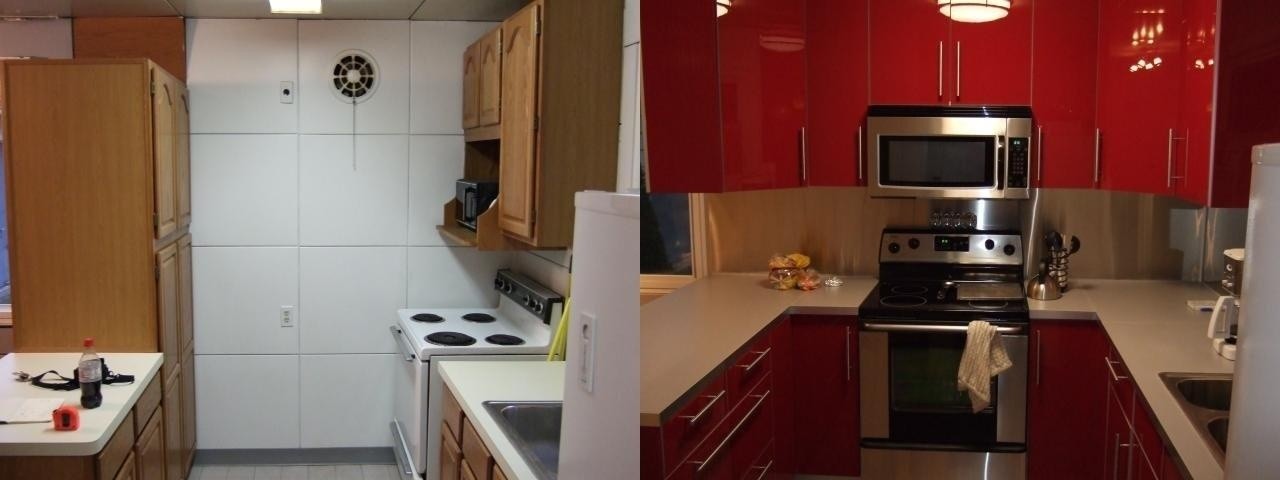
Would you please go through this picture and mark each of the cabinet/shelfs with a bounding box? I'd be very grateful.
[1,351,166,480]
[1029,0,1100,188]
[790,313,860,479]
[0,56,197,480]
[868,1,1035,104]
[1111,344,1187,479]
[1028,317,1113,479]
[809,0,869,188]
[641,313,790,479]
[641,0,811,190]
[461,27,501,140]
[438,380,511,480]
[1100,0,1280,209]
[502,1,625,252]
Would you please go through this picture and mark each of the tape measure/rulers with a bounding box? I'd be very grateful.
[52,404,80,431]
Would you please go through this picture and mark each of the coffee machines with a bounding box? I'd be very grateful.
[1208,244,1251,359]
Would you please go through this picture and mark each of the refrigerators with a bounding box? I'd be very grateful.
[554,191,640,480]
[1225,144,1279,478]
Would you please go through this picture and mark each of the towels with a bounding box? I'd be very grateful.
[957,320,1013,414]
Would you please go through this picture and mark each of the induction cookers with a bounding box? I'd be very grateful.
[859,272,1029,321]
[396,295,554,362]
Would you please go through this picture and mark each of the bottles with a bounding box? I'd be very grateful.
[78,337,102,409]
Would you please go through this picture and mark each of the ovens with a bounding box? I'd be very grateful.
[862,325,1027,480]
[392,328,559,480]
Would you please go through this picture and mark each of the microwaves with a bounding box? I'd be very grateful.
[869,107,1032,200]
[456,179,501,233]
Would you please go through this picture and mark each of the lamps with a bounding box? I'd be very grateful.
[934,1,1011,23]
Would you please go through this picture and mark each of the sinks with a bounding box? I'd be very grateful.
[1158,372,1233,415]
[481,400,562,478]
[1195,415,1229,472]
